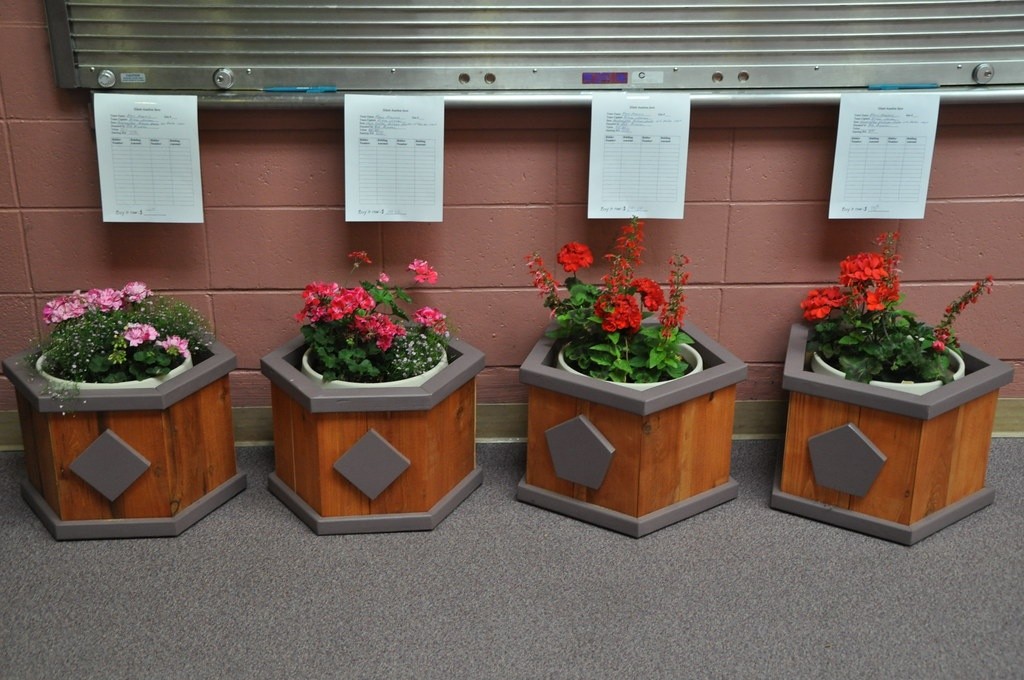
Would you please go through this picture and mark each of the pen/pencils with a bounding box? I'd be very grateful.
[259,86,339,94]
[868,82,939,90]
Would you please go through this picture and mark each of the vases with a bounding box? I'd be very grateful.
[33,329,195,391]
[557,337,705,391]
[809,336,968,396]
[299,332,448,389]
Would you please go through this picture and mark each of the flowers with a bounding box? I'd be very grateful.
[15,281,217,381]
[526,213,696,387]
[298,250,455,384]
[801,231,994,388]
